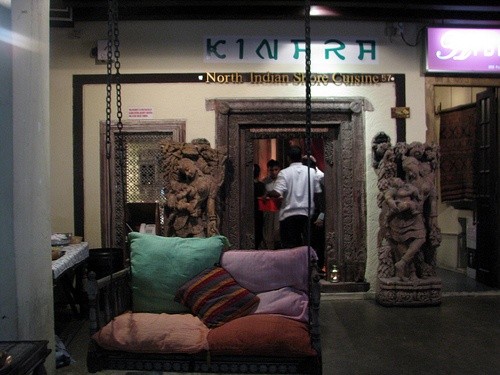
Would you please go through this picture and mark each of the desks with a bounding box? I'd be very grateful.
[52,241,89,321]
[0,340,52,375]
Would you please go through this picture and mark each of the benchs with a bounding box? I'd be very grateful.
[86,266,322,375]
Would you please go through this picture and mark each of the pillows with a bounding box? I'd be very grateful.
[175,264,260,328]
[93,311,210,354]
[128,232,230,314]
[207,315,317,357]
[221,246,318,322]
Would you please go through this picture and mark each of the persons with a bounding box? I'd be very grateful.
[254,145,326,275]
[166,158,219,237]
[386,156,436,281]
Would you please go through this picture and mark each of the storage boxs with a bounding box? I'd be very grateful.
[258,197,278,212]
